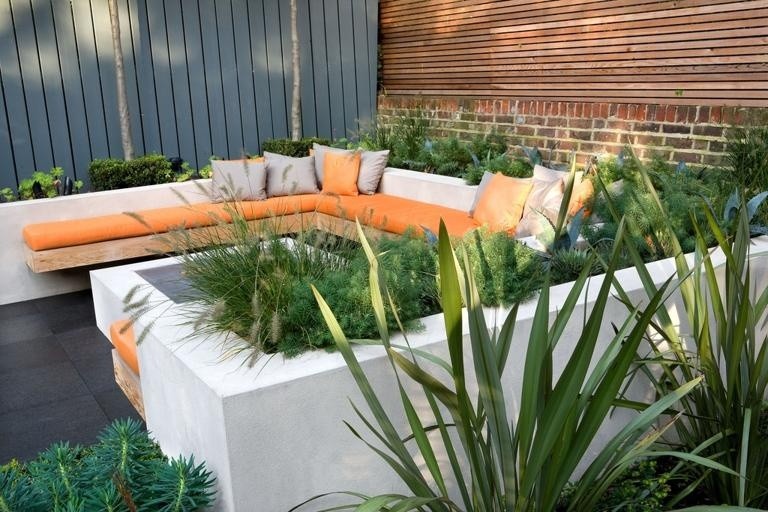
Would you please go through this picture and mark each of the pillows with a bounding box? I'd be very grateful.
[206,141,390,204]
[469,164,624,253]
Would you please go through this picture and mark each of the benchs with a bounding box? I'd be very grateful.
[19,165,505,282]
